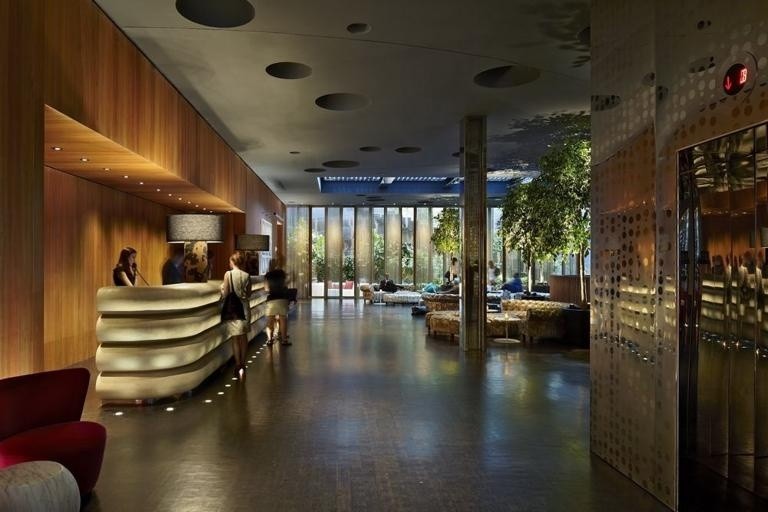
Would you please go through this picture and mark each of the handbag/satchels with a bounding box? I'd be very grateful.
[222,290,246,323]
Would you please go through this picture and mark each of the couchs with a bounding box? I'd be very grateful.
[422,292,496,312]
[360,285,426,304]
[502,299,570,344]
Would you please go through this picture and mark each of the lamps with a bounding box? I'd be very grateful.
[234,234,269,276]
[166,214,224,283]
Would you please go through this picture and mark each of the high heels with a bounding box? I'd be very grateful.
[240,360,247,374]
[232,364,241,378]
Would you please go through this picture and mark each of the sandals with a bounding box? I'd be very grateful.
[267,340,273,346]
[281,340,292,345]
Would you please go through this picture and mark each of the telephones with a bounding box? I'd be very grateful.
[132,262,138,268]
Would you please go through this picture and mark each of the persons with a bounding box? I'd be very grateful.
[488,267,502,309]
[163,247,187,285]
[111,247,141,288]
[501,273,523,293]
[220,251,252,380]
[379,273,394,292]
[449,257,459,281]
[487,260,497,285]
[265,258,293,345]
[176,252,200,282]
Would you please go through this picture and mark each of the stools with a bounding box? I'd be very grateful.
[0,461,81,512]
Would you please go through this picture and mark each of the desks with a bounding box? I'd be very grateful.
[426,310,522,344]
[383,294,421,306]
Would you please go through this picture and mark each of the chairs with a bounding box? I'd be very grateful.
[0,368,106,500]
